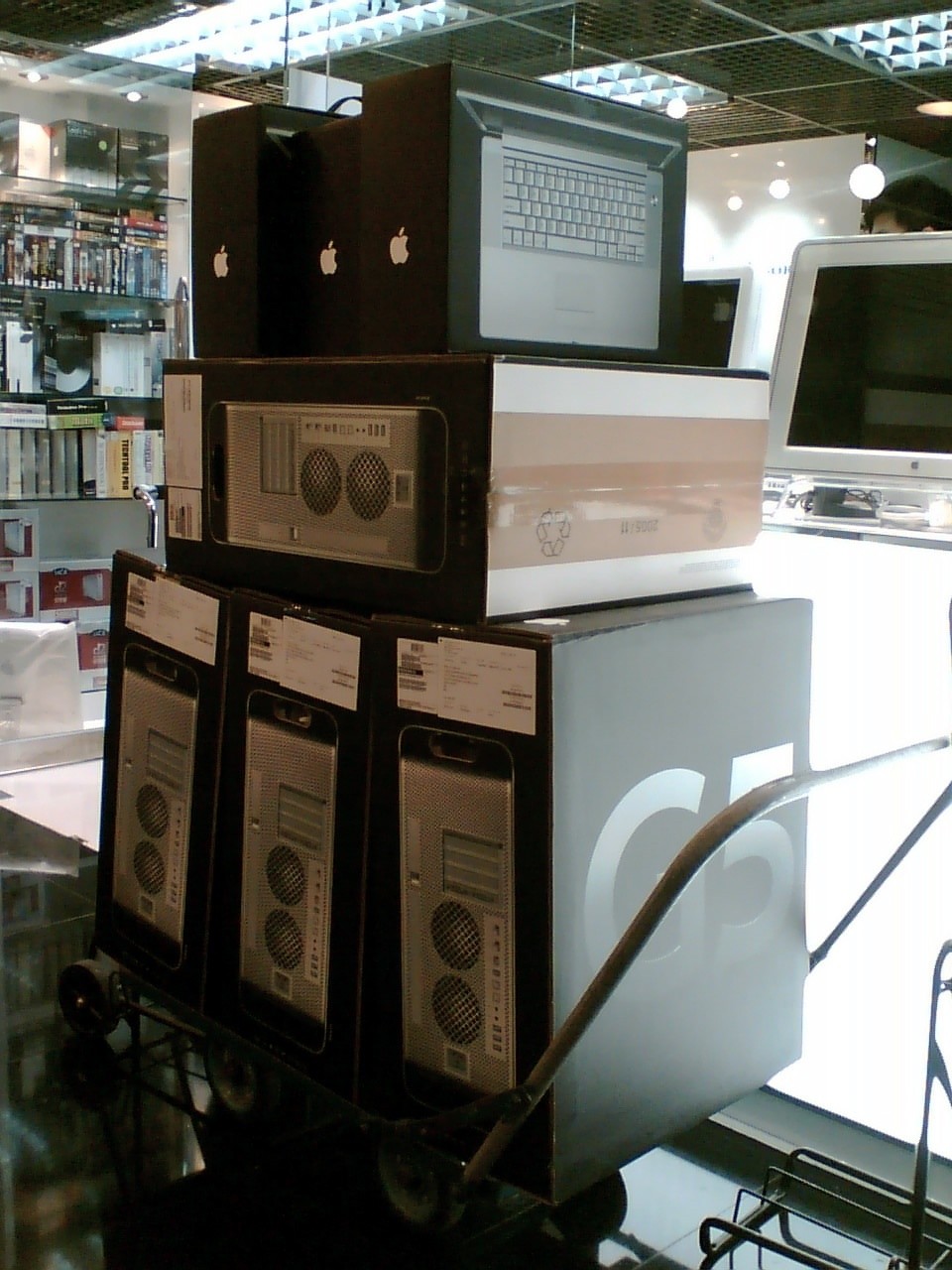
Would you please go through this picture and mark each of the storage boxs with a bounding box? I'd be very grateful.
[0,509,114,693]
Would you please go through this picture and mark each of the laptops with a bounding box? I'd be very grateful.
[455,87,683,359]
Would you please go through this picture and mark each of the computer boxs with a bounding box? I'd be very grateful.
[90,354,820,1201]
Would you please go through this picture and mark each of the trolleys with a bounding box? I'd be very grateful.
[54,735,951,1269]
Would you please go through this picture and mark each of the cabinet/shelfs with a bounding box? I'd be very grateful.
[1,175,187,504]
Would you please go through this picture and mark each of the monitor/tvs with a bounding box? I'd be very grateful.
[763,231,952,521]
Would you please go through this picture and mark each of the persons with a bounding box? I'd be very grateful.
[863,175,952,234]
